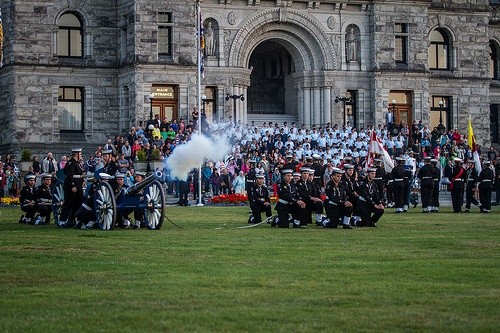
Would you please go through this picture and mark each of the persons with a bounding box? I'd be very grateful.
[0,109,500,229]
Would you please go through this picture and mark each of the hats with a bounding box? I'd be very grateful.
[394,157,406,164]
[102,149,112,155]
[114,174,125,179]
[454,158,491,165]
[99,172,111,180]
[25,173,37,181]
[306,156,312,160]
[255,173,265,179]
[367,168,377,171]
[331,164,354,173]
[424,156,438,162]
[71,148,83,152]
[312,154,324,160]
[136,170,147,176]
[261,154,266,157]
[286,156,293,159]
[249,160,255,165]
[346,157,352,161]
[42,174,53,180]
[293,168,315,176]
[282,169,294,176]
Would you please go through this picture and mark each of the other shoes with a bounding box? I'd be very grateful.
[387,202,492,214]
[18,214,141,229]
[247,214,378,229]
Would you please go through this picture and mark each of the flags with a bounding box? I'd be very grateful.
[196,8,206,80]
[468,118,482,176]
[370,130,394,172]
